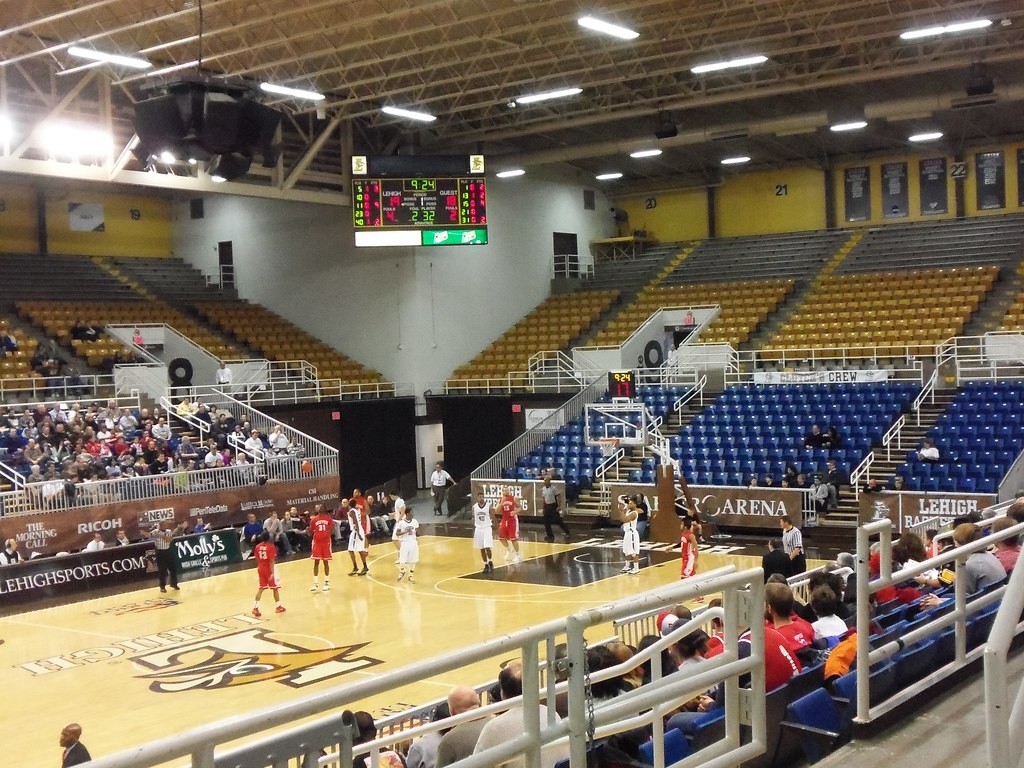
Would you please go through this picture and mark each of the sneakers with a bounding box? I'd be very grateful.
[252,608,261,616]
[628,569,639,574]
[311,584,319,591]
[322,583,329,591]
[621,567,630,572]
[276,606,285,612]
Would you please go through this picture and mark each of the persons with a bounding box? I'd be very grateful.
[336,488,1024,768]
[0,516,212,565]
[305,503,335,590]
[664,517,704,604]
[0,328,19,359]
[750,424,843,519]
[526,467,563,480]
[431,462,456,515]
[252,530,286,616]
[30,339,90,396]
[863,479,882,493]
[151,518,180,592]
[892,476,907,490]
[73,320,96,338]
[541,477,571,543]
[244,503,344,556]
[391,490,410,564]
[916,438,939,465]
[497,489,523,561]
[471,492,499,572]
[59,723,91,768]
[620,496,640,575]
[396,506,420,585]
[216,362,232,396]
[0,395,308,509]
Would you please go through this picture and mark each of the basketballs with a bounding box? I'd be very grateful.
[301,463,313,472]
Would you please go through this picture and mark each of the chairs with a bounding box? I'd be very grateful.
[0,261,1024,768]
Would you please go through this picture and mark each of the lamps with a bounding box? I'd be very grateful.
[1002,18,1012,25]
[653,100,679,139]
[507,101,515,107]
[965,61,995,97]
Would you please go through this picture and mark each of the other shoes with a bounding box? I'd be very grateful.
[484,565,489,573]
[358,568,368,575]
[171,585,180,590]
[489,565,493,572]
[397,572,405,581]
[409,577,415,583]
[348,568,358,575]
[161,587,166,592]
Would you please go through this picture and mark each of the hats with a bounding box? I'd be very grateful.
[663,619,688,635]
[657,611,679,633]
